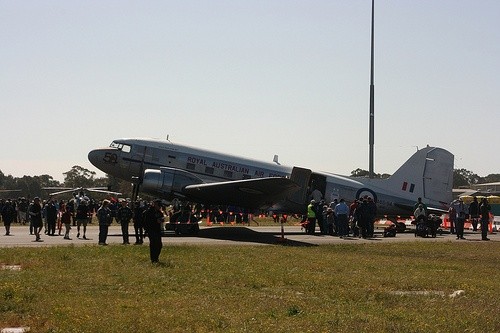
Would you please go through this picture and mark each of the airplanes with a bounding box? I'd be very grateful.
[87,134,454,224]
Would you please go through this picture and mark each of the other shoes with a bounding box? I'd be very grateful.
[134,242,139,244]
[456,236,459,239]
[45,230,62,236]
[64,236,70,239]
[123,242,130,244]
[482,237,490,241]
[77,233,80,237]
[30,232,32,234]
[99,242,107,245]
[139,241,143,245]
[460,237,466,239]
[473,229,477,231]
[36,238,41,241]
[83,236,86,239]
[6,232,10,235]
[307,230,375,239]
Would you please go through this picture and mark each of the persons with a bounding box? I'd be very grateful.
[450,198,469,240]
[478,197,490,241]
[469,197,480,231]
[141,199,166,263]
[413,197,439,238]
[448,202,458,235]
[0,185,379,245]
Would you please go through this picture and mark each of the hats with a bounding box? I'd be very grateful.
[311,200,315,203]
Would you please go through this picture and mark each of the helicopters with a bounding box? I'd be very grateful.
[41,186,124,206]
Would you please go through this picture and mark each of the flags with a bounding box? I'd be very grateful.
[401,181,415,193]
